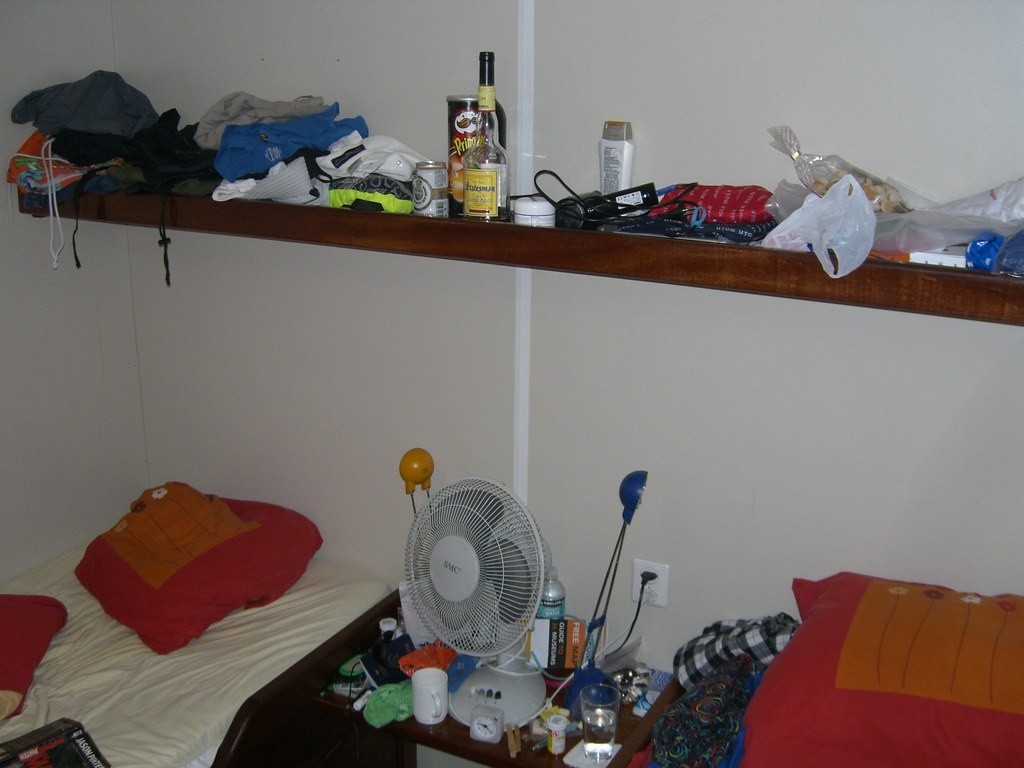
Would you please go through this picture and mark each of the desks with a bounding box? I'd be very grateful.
[313,669,681,768]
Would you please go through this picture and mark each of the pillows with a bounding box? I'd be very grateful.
[0,537,401,767]
[0,593,70,719]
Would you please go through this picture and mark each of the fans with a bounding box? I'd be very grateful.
[405,476,552,736]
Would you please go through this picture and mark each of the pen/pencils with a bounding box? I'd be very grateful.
[531,720,578,753]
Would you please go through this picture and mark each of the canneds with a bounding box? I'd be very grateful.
[412,162,449,218]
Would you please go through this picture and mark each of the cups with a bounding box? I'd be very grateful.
[581,685,621,763]
[412,668,448,725]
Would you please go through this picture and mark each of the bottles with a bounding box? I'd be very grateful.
[463,52,509,220]
[535,567,565,620]
[380,618,397,641]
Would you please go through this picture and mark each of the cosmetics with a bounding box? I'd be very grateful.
[352,688,375,713]
[515,197,555,226]
[597,121,633,196]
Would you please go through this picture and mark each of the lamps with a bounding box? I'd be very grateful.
[562,470,649,718]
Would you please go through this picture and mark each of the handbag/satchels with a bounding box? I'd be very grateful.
[760,174,877,278]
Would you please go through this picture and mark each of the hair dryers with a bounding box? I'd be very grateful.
[553,182,660,230]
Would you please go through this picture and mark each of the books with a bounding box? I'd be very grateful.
[520,618,582,671]
[0,717,112,768]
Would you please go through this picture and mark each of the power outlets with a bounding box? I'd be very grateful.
[630,559,669,607]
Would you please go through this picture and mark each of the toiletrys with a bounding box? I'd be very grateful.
[598,122,633,194]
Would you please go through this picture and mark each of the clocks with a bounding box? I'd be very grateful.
[469,705,505,745]
